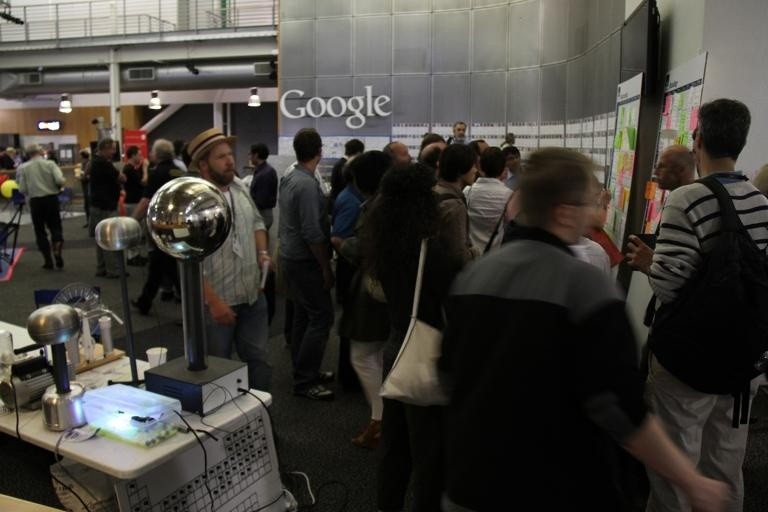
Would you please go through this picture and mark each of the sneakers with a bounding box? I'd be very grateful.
[313,369,334,381]
[293,382,335,400]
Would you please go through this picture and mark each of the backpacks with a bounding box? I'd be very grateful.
[644,177,767,395]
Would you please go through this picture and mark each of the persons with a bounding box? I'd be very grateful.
[432,143,740,510]
[186,127,767,356]
[446,121,470,145]
[1,139,190,312]
[352,161,462,511]
[623,96,766,510]
[326,149,411,453]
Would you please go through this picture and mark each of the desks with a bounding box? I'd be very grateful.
[1,319,280,511]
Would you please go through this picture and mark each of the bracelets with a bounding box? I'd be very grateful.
[257,248,268,256]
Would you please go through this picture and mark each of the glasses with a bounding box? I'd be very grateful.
[591,183,607,206]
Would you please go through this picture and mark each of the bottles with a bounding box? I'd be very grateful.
[97,315,112,358]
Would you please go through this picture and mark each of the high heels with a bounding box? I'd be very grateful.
[351,420,383,448]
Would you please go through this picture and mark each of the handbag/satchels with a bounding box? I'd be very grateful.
[377,317,455,408]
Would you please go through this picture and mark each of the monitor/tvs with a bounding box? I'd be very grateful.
[619,0,660,98]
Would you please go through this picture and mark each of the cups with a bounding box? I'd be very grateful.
[145,347,168,368]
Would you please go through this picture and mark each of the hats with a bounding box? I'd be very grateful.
[187,128,239,174]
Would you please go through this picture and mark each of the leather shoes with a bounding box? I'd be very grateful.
[131,296,150,315]
[126,257,144,266]
[161,291,175,300]
[106,271,130,278]
[137,255,148,262]
[54,250,63,268]
[97,271,106,276]
[42,264,53,271]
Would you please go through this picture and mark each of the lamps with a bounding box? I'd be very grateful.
[58,93,71,113]
[246,87,260,107]
[148,90,162,109]
